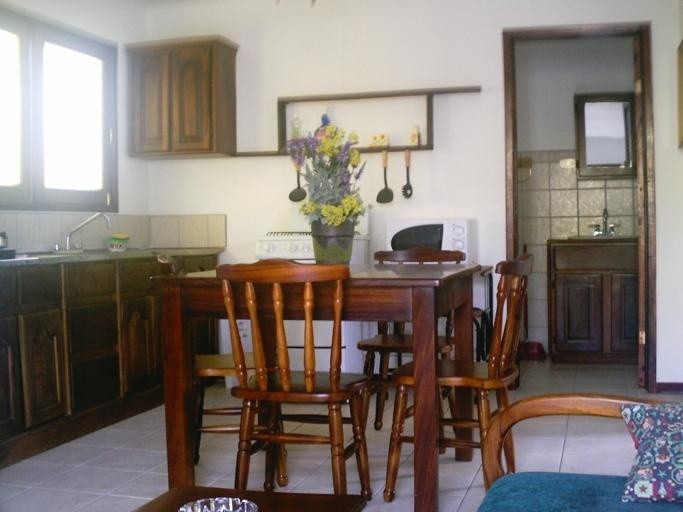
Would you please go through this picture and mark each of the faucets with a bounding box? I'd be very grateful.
[602,209,609,236]
[65,209,112,251]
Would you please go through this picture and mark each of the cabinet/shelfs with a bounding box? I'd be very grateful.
[1,263,72,464]
[546,240,640,364]
[65,261,123,435]
[122,255,220,421]
[123,36,241,159]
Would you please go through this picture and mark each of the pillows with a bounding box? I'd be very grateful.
[620,403,683,505]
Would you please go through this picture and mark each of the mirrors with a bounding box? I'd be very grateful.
[573,92,636,180]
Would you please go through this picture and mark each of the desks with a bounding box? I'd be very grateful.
[143,262,486,512]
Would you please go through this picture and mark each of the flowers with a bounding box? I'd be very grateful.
[290,123,372,224]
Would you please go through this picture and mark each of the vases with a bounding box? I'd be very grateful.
[308,213,357,267]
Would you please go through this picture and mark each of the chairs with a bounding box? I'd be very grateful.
[474,391,683,512]
